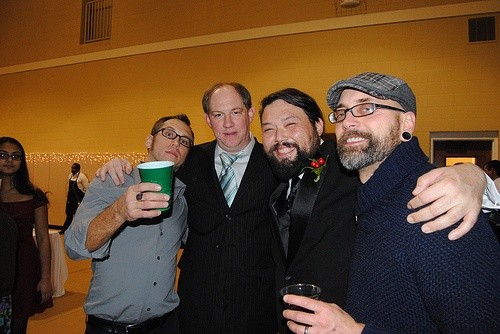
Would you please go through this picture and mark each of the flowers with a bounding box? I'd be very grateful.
[301,154,330,182]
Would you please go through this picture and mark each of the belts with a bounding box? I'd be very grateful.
[88,311,173,334]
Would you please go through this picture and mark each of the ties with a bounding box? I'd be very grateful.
[219,153,239,208]
[285,175,301,216]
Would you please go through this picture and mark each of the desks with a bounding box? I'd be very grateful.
[33,229,61,296]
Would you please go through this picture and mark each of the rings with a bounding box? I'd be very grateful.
[136,192,143,200]
[304,325,308,334]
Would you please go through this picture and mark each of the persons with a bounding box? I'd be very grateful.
[281,74,500,334]
[258,87,500,334]
[0,138,53,334]
[59,164,91,235]
[67,114,195,334]
[483,159,500,195]
[96,83,260,334]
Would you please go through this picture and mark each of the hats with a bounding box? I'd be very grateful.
[327,72,416,116]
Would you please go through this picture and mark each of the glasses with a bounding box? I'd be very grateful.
[328,103,407,123]
[0,153,21,160]
[153,128,193,148]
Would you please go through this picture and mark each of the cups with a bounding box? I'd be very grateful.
[137,161,175,212]
[280,283,322,327]
